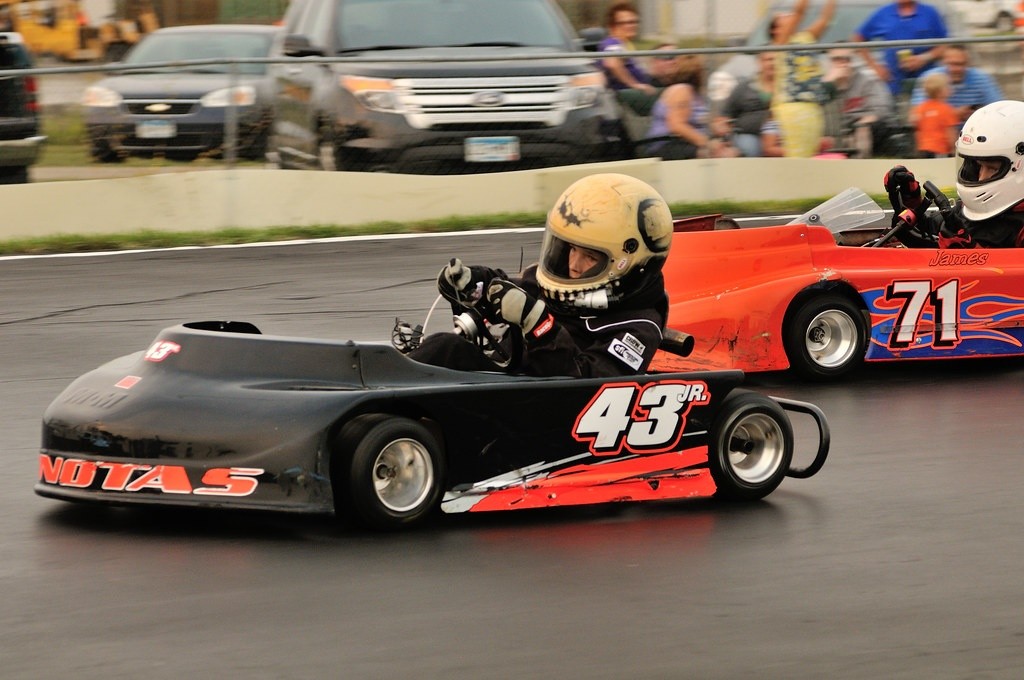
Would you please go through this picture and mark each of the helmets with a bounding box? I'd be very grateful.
[536,174,673,303]
[955,98,1024,223]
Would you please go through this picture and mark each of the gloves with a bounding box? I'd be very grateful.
[437,257,482,308]
[487,276,544,338]
[939,219,974,250]
[885,166,920,205]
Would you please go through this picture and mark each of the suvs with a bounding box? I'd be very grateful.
[270,1,637,176]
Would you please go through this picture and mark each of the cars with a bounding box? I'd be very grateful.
[78,22,281,163]
[962,0,1023,35]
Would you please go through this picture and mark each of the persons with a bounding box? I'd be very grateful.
[596,1,998,159]
[884,100,1024,248]
[405,174,673,379]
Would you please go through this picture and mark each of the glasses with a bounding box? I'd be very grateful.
[612,19,640,24]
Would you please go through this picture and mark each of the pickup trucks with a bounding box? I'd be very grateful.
[2,30,50,186]
